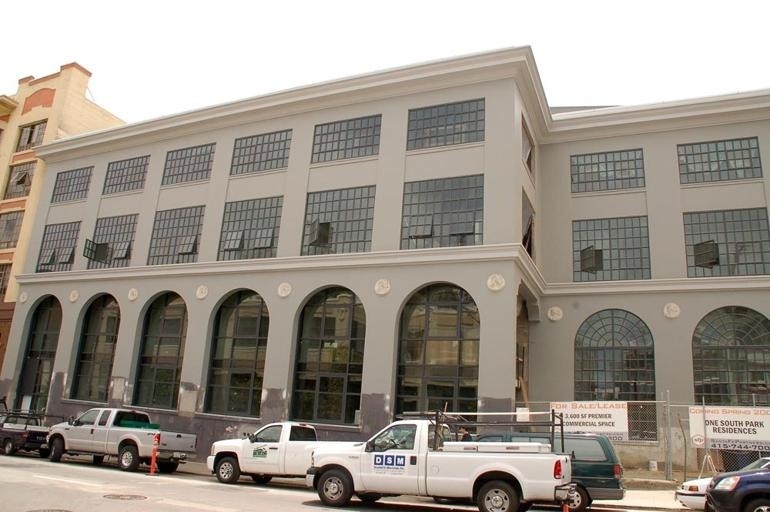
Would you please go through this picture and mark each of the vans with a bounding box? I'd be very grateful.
[466,430,623,512]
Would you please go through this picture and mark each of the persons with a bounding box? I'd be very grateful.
[459,426,472,441]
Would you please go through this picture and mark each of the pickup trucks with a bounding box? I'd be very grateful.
[45,408,197,472]
[207,421,366,485]
[307,414,577,512]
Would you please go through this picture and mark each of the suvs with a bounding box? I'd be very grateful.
[0,408,50,458]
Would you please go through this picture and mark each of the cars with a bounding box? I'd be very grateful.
[676,458,770,512]
